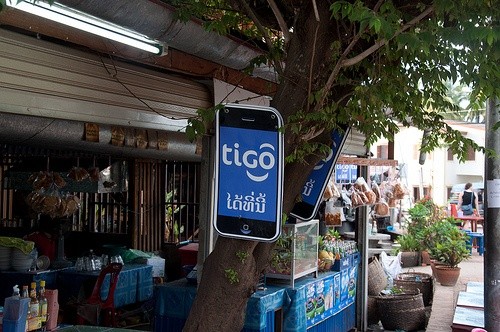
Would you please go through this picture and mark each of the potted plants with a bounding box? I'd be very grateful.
[397,198,469,286]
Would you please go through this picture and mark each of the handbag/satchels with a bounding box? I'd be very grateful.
[461,204,473,216]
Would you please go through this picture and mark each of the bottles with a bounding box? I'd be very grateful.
[13,280,48,332]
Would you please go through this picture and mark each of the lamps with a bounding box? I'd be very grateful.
[6,0,168,56]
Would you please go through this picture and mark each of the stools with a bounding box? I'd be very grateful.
[462,230,483,256]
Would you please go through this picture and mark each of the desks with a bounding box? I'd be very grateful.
[455,217,485,248]
[0,257,365,332]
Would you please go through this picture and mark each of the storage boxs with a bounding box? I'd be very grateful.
[332,252,361,271]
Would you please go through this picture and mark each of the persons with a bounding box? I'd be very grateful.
[23,214,60,259]
[456,183,480,228]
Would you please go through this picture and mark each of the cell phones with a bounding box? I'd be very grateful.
[213,102,283,243]
[288,118,354,222]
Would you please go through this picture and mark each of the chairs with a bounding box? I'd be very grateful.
[65,262,122,326]
